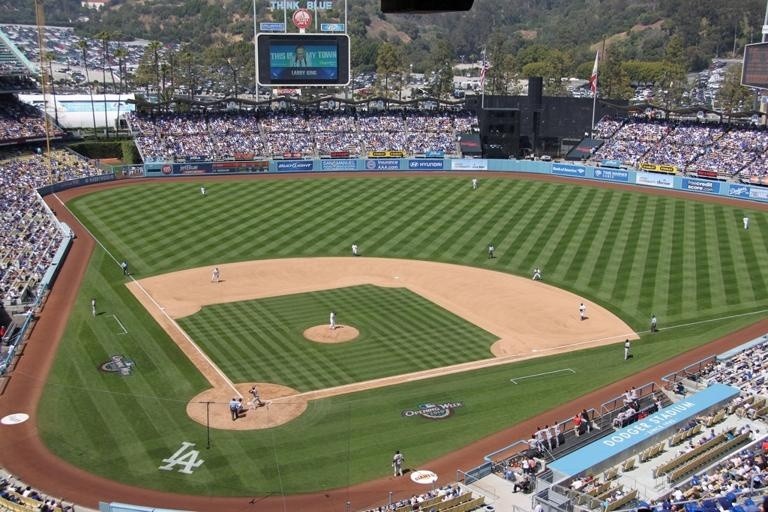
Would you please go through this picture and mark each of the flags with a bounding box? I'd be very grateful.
[480,51,494,87]
[587,53,601,95]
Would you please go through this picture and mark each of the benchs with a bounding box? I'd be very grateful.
[394,490,486,511]
[567,342,768,512]
[0,486,64,512]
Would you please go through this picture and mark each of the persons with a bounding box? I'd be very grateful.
[330,311,337,329]
[578,303,585,321]
[472,177,478,190]
[531,267,543,281]
[590,115,767,196]
[211,267,220,282]
[237,398,244,414]
[487,244,496,259]
[742,216,749,230]
[200,186,205,196]
[1,468,76,511]
[351,241,358,255]
[229,398,239,421]
[292,46,311,68]
[121,260,128,275]
[248,384,263,411]
[650,315,656,332]
